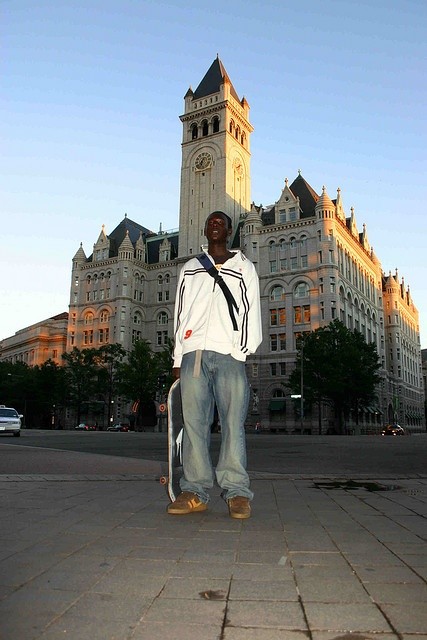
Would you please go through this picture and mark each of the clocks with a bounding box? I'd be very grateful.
[194,151,213,172]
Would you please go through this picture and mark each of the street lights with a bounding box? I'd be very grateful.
[7,374,27,428]
[108,400,114,427]
[145,340,171,395]
[301,328,331,434]
[53,404,56,429]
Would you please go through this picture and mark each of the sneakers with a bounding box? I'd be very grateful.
[167,491,209,514]
[229,495,251,519]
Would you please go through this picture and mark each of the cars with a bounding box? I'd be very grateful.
[75,423,96,430]
[107,423,128,432]
[0,405,23,436]
[380,424,404,436]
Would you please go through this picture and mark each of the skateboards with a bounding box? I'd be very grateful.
[159,378,184,502]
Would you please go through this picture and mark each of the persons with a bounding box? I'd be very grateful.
[166,210,262,519]
